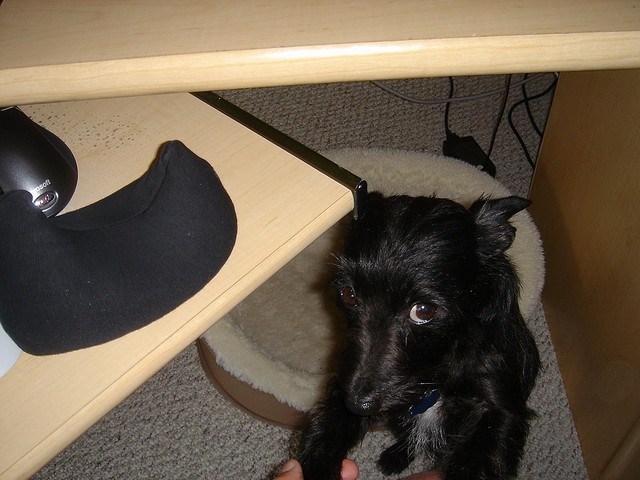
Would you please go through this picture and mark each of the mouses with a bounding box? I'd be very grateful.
[0,106,78,219]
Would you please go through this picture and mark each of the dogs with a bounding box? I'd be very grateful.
[298,190,543,480]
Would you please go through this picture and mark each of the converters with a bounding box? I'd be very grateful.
[442,136,496,180]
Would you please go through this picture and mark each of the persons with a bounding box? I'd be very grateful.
[271,458,359,479]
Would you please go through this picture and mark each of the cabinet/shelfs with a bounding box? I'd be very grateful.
[0,0,639,479]
